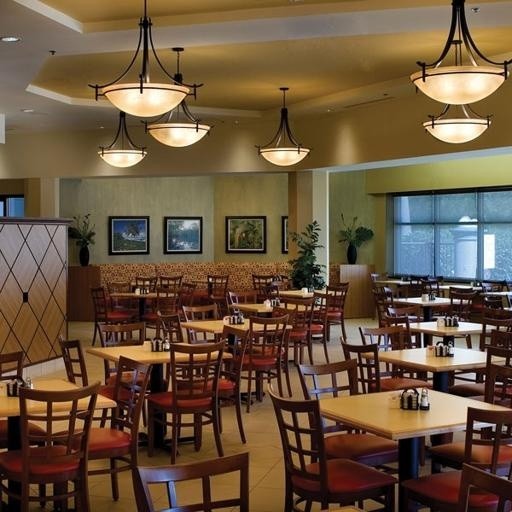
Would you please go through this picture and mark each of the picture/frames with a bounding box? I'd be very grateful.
[280,213,289,255]
[162,214,203,255]
[225,215,268,254]
[106,214,150,256]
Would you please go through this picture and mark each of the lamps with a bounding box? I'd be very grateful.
[146,46,216,148]
[256,88,313,167]
[96,108,147,168]
[90,0,204,120]
[406,2,512,103]
[420,98,492,146]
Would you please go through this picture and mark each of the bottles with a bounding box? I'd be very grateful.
[142,334,172,353]
[388,386,434,413]
[434,313,464,329]
[224,307,247,326]
[262,298,283,308]
[426,340,455,359]
[6,375,36,398]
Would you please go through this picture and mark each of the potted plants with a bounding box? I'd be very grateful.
[68,214,96,265]
[336,211,374,265]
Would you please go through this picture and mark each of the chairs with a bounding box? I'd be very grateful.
[1,273,512,512]
